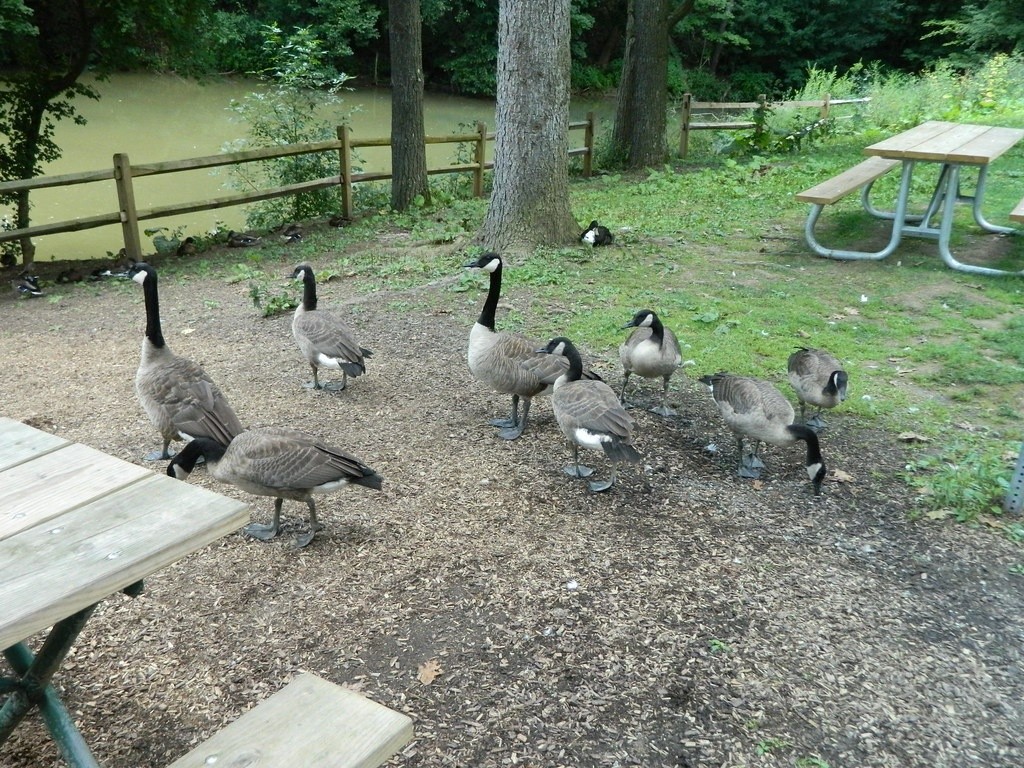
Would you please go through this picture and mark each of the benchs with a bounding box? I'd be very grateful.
[161,672,415,768]
[795,155,904,205]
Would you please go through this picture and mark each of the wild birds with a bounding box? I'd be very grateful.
[165,426,383,550]
[285,264,373,392]
[101,262,243,468]
[580,220,614,248]
[459,252,609,440]
[618,308,684,417]
[698,372,826,500]
[534,336,647,493]
[786,345,849,432]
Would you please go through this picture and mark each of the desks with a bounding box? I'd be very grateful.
[0,415,252,767]
[795,119,1024,279]
